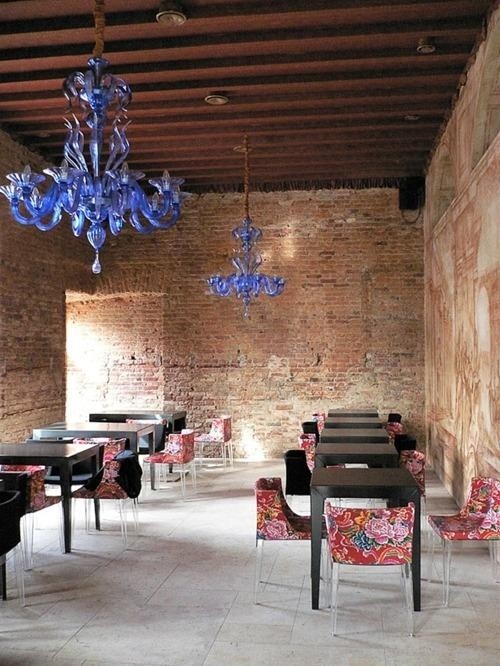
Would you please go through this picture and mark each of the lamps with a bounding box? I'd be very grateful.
[2,1,184,279]
[207,135,286,319]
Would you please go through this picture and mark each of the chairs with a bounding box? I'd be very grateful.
[143,429,198,498]
[425,471,498,607]
[192,416,234,470]
[255,476,327,609]
[382,406,428,495]
[323,499,417,639]
[0,463,67,571]
[67,436,141,545]
[282,407,342,510]
[0,490,27,607]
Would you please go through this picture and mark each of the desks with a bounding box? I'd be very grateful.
[0,442,101,553]
[316,406,402,505]
[30,407,189,505]
[309,467,424,612]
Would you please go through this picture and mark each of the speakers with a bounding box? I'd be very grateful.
[399,177,425,209]
